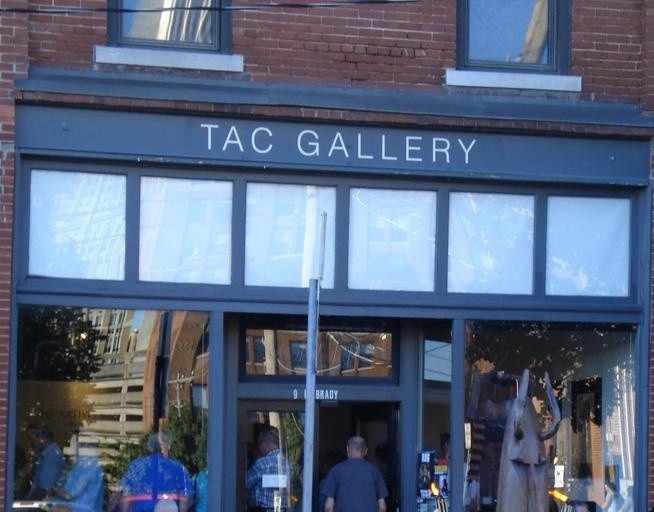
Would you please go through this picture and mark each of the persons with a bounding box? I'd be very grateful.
[26,423,104,512]
[107,432,208,512]
[601,483,633,512]
[319,436,388,512]
[245,427,295,512]
